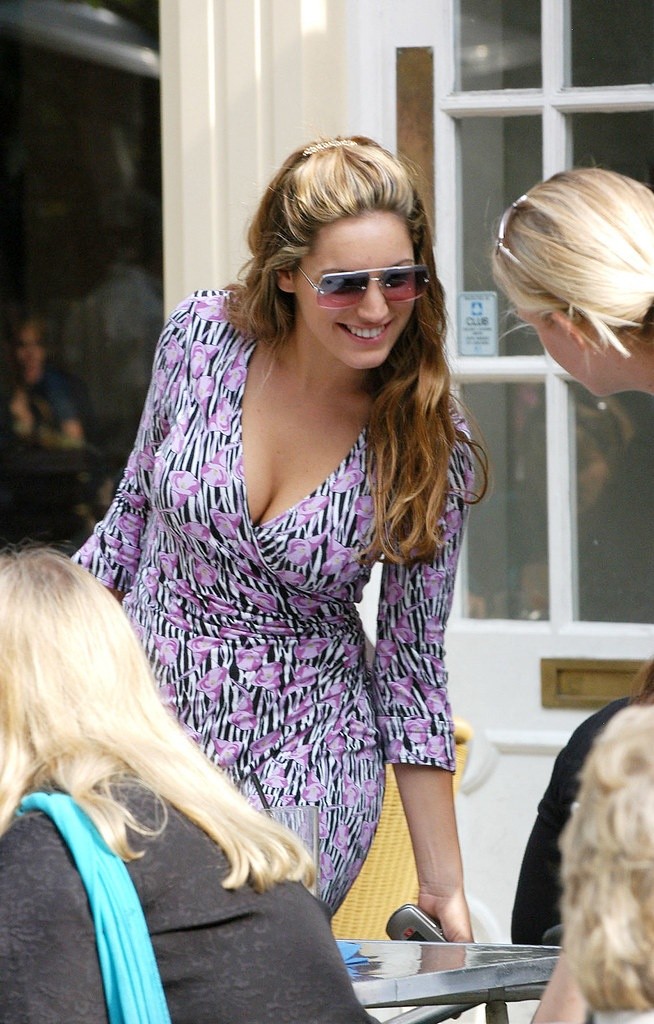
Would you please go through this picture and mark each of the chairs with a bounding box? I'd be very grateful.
[330,722,470,938]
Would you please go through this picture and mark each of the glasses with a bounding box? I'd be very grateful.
[293,251,431,310]
[492,195,585,326]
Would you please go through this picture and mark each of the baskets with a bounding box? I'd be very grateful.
[331,711,472,939]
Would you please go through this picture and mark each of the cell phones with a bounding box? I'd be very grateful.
[385,904,448,942]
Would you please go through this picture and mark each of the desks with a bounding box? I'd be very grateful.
[336,938,562,1024]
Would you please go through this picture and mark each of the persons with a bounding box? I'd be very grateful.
[494,167,654,393]
[513,384,603,509]
[530,704,654,1024]
[511,662,654,946]
[0,547,379,1024]
[0,306,115,548]
[68,137,473,942]
